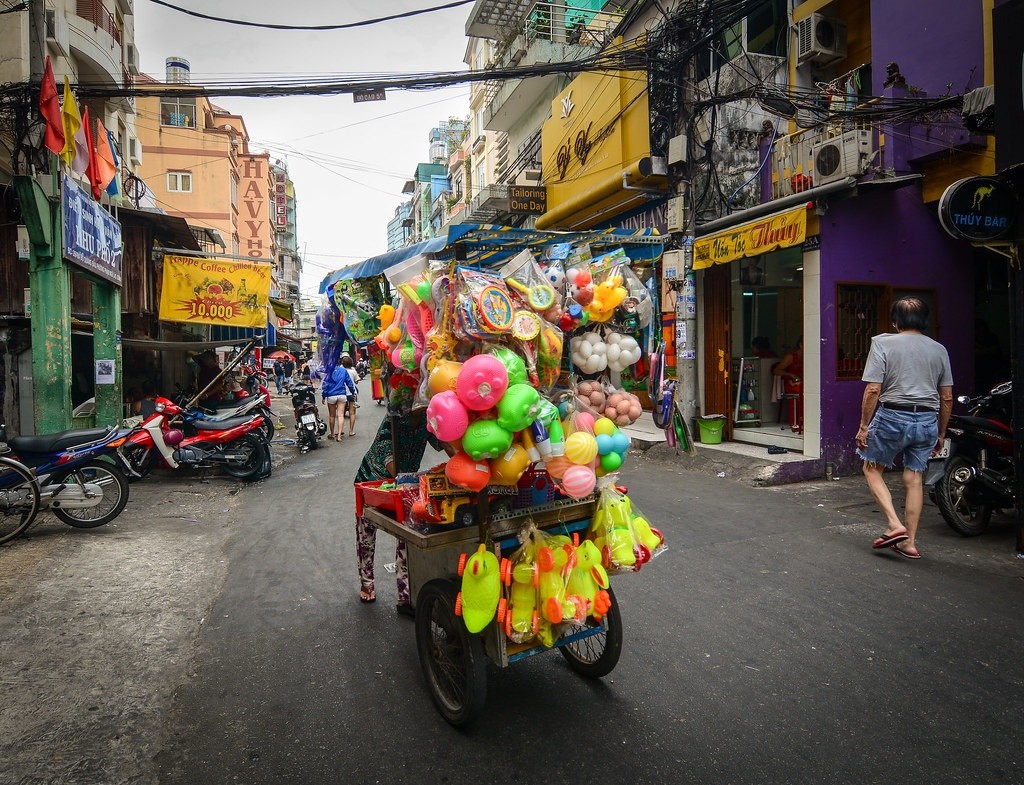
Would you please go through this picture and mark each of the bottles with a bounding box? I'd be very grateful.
[666,427,676,447]
[732,359,758,420]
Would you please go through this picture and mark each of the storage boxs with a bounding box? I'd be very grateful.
[73,416,95,429]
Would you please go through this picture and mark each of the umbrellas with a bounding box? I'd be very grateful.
[265,350,296,363]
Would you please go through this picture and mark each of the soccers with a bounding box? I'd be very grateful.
[543,267,565,290]
[567,283,581,298]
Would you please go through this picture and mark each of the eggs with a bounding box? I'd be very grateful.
[569,332,641,374]
[577,381,643,425]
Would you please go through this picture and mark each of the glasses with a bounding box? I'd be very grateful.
[892,321,896,328]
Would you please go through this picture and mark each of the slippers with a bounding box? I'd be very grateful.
[396,603,416,618]
[872,533,910,549]
[889,543,922,559]
[360,586,377,603]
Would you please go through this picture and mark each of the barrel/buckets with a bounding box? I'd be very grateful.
[697,420,724,445]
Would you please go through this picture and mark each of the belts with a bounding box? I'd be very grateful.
[882,401,936,412]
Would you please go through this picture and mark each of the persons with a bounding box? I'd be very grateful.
[187,350,225,410]
[335,351,365,436]
[353,394,455,619]
[772,332,803,428]
[321,355,356,442]
[853,295,954,560]
[272,354,294,395]
[301,357,310,384]
[132,379,164,421]
[225,376,271,408]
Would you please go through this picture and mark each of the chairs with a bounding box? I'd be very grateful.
[771,362,798,425]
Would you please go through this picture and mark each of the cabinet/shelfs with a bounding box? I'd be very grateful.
[732,356,762,428]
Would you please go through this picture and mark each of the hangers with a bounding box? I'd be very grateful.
[815,67,861,94]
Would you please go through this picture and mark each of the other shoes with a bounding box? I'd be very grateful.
[336,437,344,442]
[349,432,356,436]
[328,434,336,440]
[335,432,345,435]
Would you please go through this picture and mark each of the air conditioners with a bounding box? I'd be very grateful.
[812,130,872,187]
[798,12,848,63]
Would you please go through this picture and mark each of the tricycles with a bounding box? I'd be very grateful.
[361,491,626,715]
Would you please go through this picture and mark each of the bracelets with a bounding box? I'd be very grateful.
[203,392,210,398]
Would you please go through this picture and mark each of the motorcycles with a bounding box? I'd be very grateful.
[0,425,129,544]
[111,390,273,481]
[289,385,327,451]
[246,358,268,388]
[923,378,1024,534]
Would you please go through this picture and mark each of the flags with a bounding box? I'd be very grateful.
[39,55,120,201]
